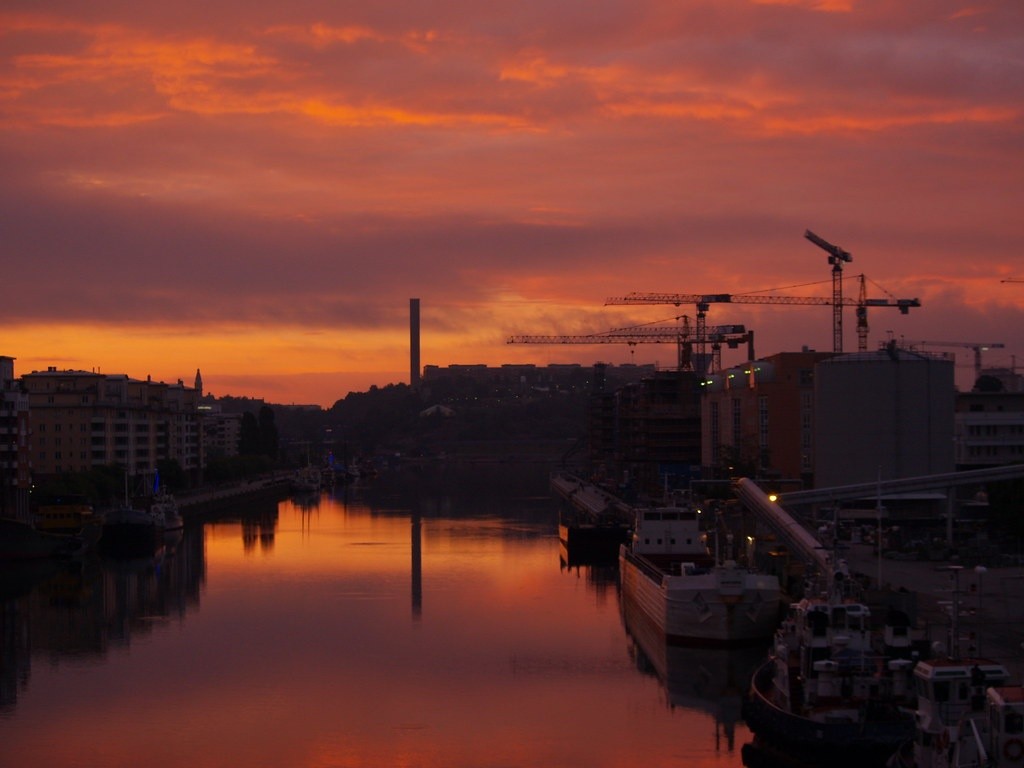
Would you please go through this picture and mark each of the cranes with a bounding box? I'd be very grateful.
[805,228,853,354]
[506,313,755,374]
[878,340,1005,383]
[605,273,926,352]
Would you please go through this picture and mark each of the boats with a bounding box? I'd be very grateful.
[291,462,321,492]
[117,471,183,528]
[620,504,782,645]
[749,593,921,765]
[874,565,1024,768]
